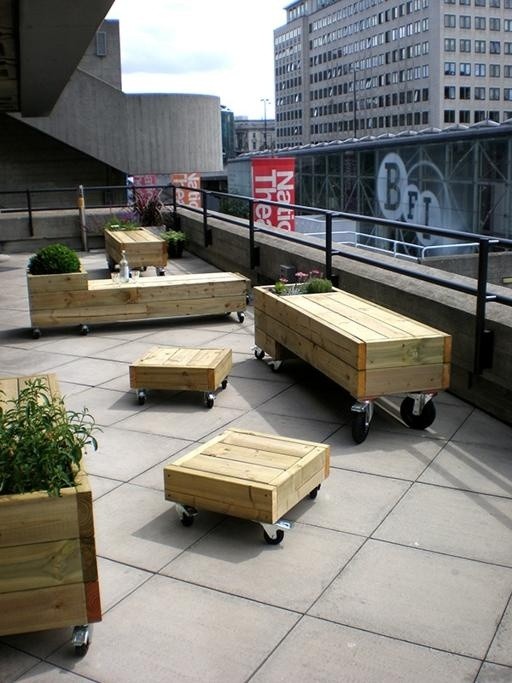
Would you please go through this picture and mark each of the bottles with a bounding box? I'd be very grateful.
[120,251,129,283]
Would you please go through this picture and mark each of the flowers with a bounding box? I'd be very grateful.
[274,270,333,294]
[103,209,141,230]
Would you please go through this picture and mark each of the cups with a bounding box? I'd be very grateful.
[130,271,139,284]
[111,272,120,286]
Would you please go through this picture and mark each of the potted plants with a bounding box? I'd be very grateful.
[0,373,104,657]
[26,243,88,291]
[159,228,187,258]
